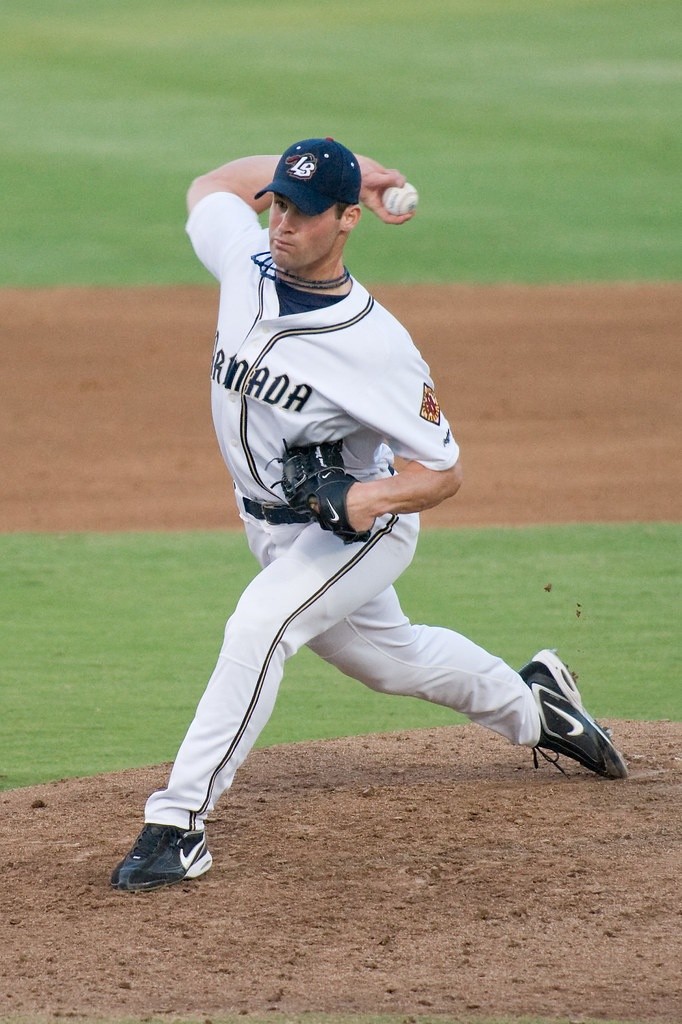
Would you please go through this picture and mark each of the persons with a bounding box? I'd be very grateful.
[111,141,632,895]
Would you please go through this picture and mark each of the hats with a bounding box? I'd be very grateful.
[252,135,363,216]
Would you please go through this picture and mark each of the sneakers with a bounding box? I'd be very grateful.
[111,822,213,890]
[514,648,628,782]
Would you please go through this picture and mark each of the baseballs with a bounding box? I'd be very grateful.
[382,180,419,216]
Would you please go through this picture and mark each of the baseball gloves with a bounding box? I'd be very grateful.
[263,439,371,547]
